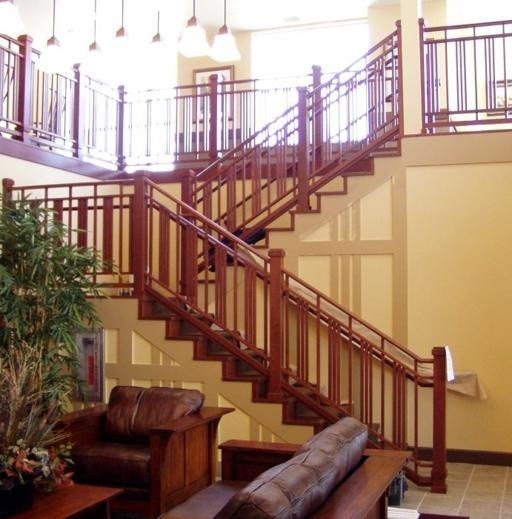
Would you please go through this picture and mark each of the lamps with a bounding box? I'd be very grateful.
[0,0,240,79]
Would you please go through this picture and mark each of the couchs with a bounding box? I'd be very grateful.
[46,397,235,518]
[156,438,414,519]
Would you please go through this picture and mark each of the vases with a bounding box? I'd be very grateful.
[0,477,34,519]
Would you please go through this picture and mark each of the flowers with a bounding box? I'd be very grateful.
[0,438,78,493]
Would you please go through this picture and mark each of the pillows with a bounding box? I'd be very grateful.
[103,385,204,443]
[212,417,369,519]
